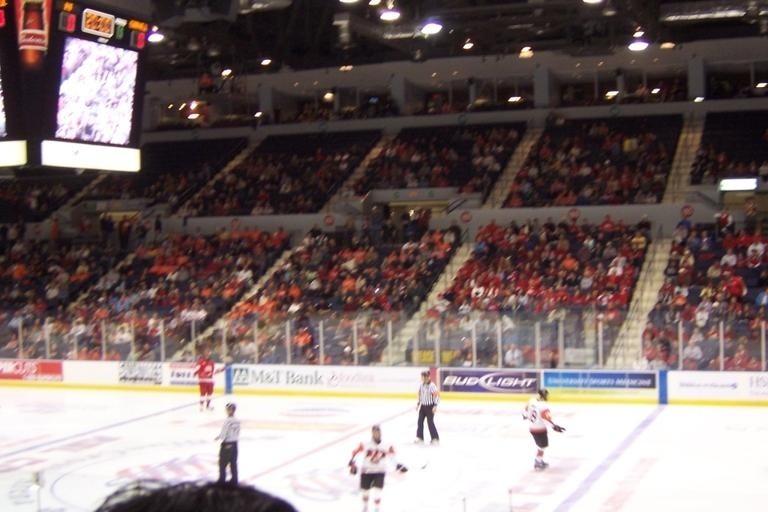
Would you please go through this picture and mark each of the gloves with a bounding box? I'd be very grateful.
[552,425,566,432]
[395,463,408,474]
[348,461,359,474]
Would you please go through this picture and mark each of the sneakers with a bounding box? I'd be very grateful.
[534,458,549,470]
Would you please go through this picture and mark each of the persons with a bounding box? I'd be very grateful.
[346,425,394,512]
[214,404,240,485]
[413,373,441,444]
[1,122,766,370]
[522,389,566,470]
[88,473,296,512]
[192,350,225,411]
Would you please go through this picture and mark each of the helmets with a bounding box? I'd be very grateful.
[372,424,380,433]
[226,402,236,410]
[537,387,549,394]
[421,370,431,377]
